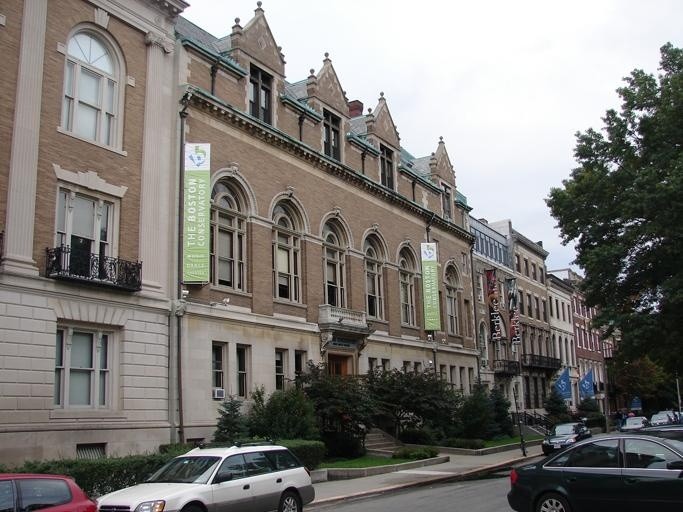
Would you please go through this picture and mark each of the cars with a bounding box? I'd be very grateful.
[507,410,683,512]
[0,473,97,512]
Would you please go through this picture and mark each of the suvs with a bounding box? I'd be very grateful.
[95,440,316,512]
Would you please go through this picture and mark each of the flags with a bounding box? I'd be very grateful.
[554,368,571,400]
[579,369,595,400]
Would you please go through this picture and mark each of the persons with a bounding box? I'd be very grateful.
[618,410,635,427]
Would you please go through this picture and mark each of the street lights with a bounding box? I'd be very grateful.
[432,342,438,381]
